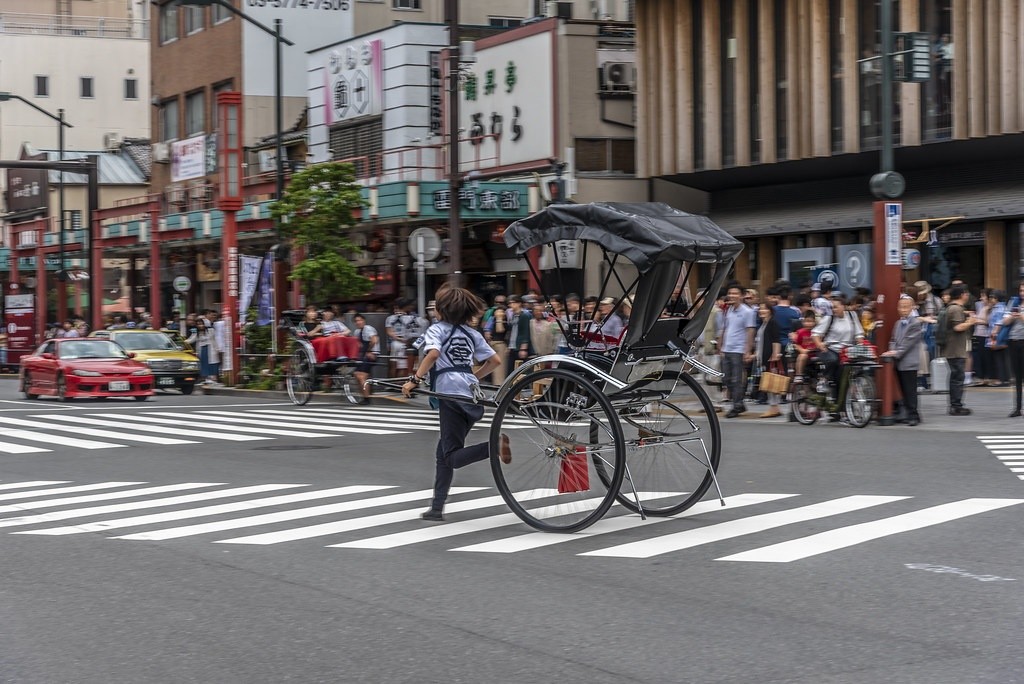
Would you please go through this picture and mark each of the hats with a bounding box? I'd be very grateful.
[914,281,932,295]
[829,291,841,296]
[811,283,824,293]
[600,297,617,305]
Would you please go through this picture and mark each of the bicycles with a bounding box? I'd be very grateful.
[786,340,886,429]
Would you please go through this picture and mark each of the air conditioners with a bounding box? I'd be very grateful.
[601,61,637,91]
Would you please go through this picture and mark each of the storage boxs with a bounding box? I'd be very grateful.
[838,345,877,365]
[758,371,790,395]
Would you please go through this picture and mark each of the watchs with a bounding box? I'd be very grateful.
[409,376,419,385]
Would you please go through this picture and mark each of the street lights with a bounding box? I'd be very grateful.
[0,91,75,331]
[151,1,295,264]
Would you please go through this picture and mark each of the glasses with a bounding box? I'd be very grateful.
[506,301,519,305]
[495,301,506,305]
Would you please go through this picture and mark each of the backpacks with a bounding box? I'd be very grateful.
[935,304,959,346]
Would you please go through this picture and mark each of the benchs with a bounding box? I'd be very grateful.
[505,316,698,421]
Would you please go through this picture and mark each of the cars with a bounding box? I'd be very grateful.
[87,328,203,397]
[17,337,157,402]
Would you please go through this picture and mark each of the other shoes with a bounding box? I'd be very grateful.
[423,510,442,521]
[499,434,511,464]
[910,418,920,426]
[759,411,781,417]
[726,405,746,418]
[949,407,969,415]
[1010,410,1021,417]
[794,375,804,383]
[816,381,827,394]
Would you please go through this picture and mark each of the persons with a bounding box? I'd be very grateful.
[879,298,918,423]
[692,277,884,422]
[161,309,224,386]
[386,297,437,397]
[45,314,92,338]
[471,289,653,417]
[352,313,380,404]
[104,312,153,330]
[902,281,1024,417]
[667,286,689,316]
[301,306,360,360]
[402,287,511,519]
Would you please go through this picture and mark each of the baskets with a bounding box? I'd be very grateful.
[845,345,879,359]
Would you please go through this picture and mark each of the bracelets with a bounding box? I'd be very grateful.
[416,374,425,380]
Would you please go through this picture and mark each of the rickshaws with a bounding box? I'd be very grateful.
[277,308,410,404]
[358,203,745,537]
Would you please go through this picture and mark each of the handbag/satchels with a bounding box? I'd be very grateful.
[759,359,791,395]
